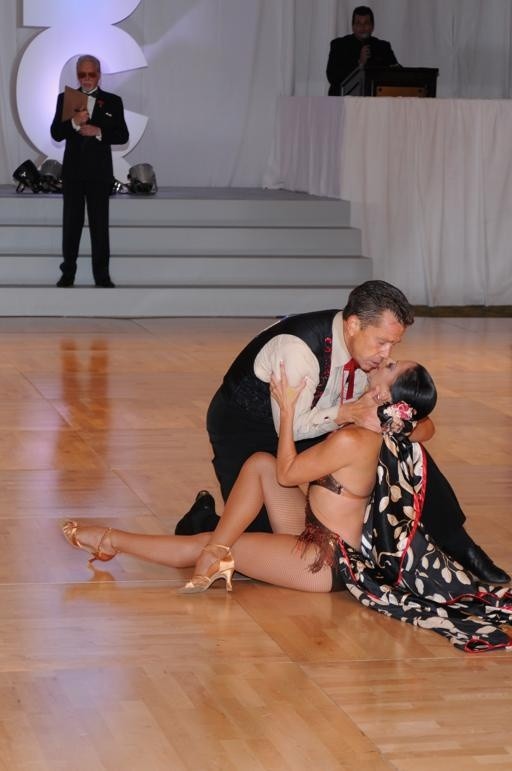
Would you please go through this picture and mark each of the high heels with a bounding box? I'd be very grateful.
[58,519,120,563]
[170,543,235,594]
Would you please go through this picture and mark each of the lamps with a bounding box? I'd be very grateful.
[12,159,157,195]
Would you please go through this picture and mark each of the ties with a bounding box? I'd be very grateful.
[342,359,359,400]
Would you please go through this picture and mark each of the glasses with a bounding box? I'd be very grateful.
[78,72,96,78]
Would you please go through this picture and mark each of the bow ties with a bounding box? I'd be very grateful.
[83,91,98,98]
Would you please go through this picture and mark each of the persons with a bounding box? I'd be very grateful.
[58,359,512,654]
[50,55,129,289]
[175,279,415,536]
[326,5,403,96]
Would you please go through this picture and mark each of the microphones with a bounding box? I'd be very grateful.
[362,32,369,46]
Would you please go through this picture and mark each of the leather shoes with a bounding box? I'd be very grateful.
[176,490,215,535]
[444,545,511,583]
[56,273,116,288]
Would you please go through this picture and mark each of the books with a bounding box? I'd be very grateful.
[62,85,88,128]
